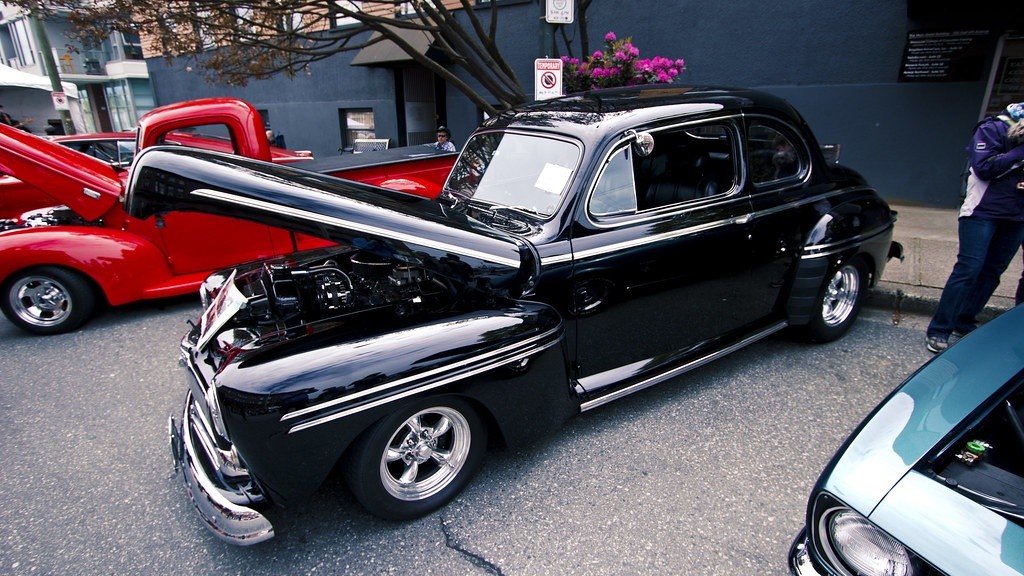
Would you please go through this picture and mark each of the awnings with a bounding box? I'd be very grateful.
[350,13,454,66]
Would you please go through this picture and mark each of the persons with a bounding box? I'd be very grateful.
[0,105,13,126]
[423,126,456,152]
[772,133,800,180]
[925,102,1024,353]
[695,151,718,197]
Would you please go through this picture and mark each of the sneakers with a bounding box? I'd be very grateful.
[925,336,949,352]
[951,325,977,337]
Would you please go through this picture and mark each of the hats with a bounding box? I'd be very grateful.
[435,125,449,132]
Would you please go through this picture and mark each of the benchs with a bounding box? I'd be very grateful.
[338,139,392,154]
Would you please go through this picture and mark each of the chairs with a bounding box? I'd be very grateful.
[603,147,720,207]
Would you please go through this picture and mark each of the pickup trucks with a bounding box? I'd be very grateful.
[0,98,460,339]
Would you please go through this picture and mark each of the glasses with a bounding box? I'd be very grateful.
[437,135,447,137]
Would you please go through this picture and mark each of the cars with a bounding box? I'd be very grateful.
[123,87,902,548]
[0,130,314,176]
[789,302,1023,576]
